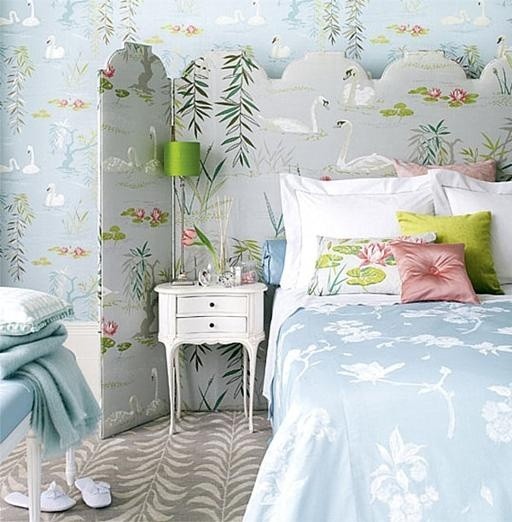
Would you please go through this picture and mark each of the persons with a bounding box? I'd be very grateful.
[201,272,207,283]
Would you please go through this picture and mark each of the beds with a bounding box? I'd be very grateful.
[242,234,511,522]
[0,349,77,522]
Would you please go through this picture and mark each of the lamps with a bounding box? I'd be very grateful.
[162,141,201,286]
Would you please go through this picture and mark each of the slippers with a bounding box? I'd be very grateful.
[4,481,76,511]
[75,476,111,508]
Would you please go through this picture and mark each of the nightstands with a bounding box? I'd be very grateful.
[154,281,267,435]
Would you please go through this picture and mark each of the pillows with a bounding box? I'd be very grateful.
[279,172,434,292]
[396,209,506,296]
[0,287,75,338]
[306,231,438,295]
[428,169,512,285]
[391,241,481,306]
[392,156,497,183]
